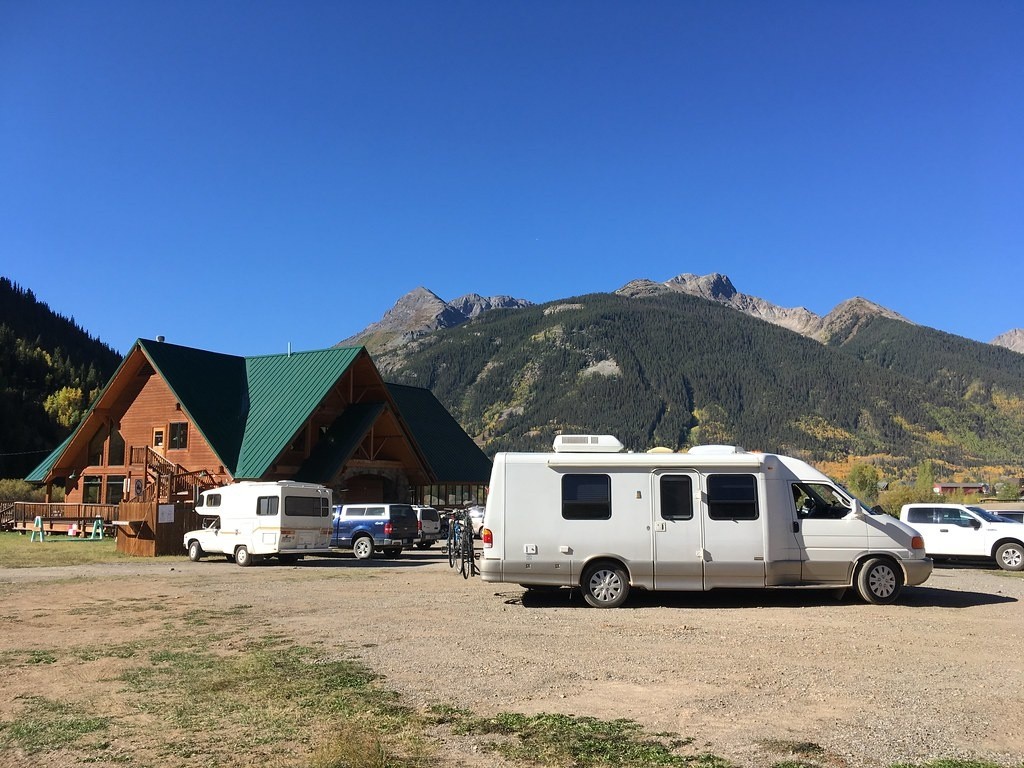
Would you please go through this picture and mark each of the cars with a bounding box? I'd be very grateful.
[438,507,484,540]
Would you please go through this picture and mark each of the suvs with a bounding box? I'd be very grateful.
[900,504,1024,571]
[408,505,441,549]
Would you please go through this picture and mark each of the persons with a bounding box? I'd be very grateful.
[793,484,801,503]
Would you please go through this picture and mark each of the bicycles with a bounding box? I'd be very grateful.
[445,507,477,580]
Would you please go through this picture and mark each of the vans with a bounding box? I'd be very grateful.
[182,479,336,566]
[478,435,933,609]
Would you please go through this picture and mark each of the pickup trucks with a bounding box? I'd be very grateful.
[330,503,417,561]
